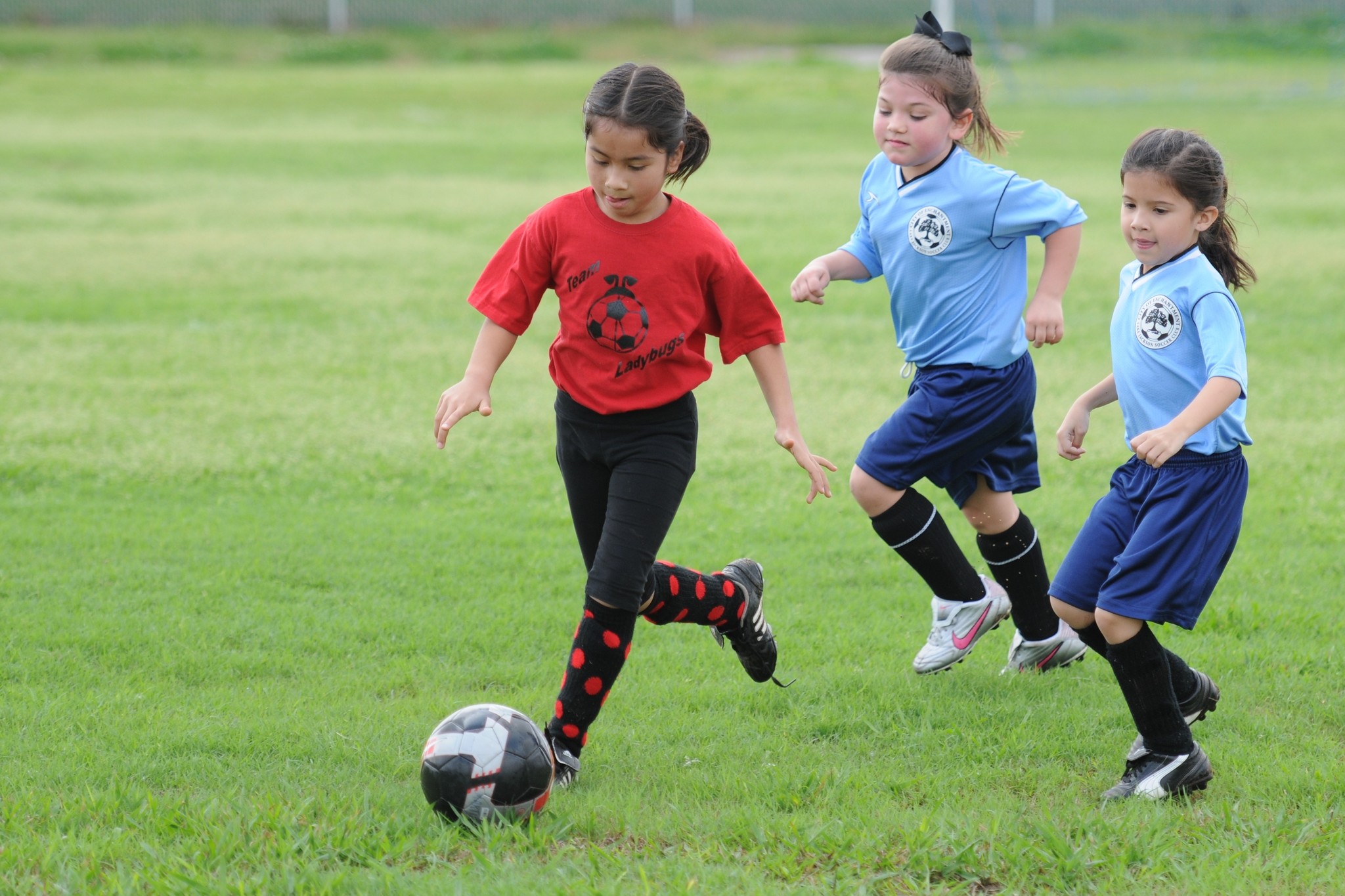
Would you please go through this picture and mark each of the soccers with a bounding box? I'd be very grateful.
[419,703,556,837]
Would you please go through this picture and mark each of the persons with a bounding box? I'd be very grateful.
[790,11,1094,673]
[1055,130,1261,804]
[433,64,837,791]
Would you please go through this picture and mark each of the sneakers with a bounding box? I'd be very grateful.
[1126,665,1221,773]
[542,722,581,790]
[999,620,1089,679]
[914,575,1014,675]
[1101,741,1215,806]
[709,558,797,689]
